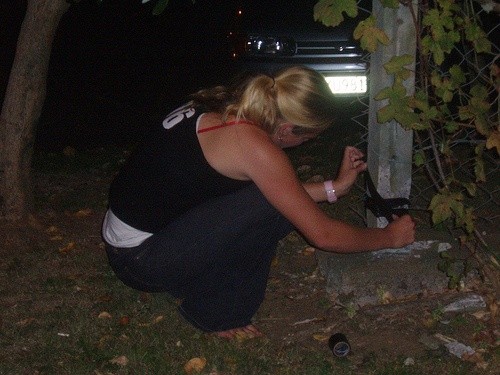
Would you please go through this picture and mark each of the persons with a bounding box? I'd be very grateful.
[99,66,417,340]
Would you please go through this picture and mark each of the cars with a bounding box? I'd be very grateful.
[233,1,425,107]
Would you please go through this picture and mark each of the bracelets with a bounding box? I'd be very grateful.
[324,179,338,204]
